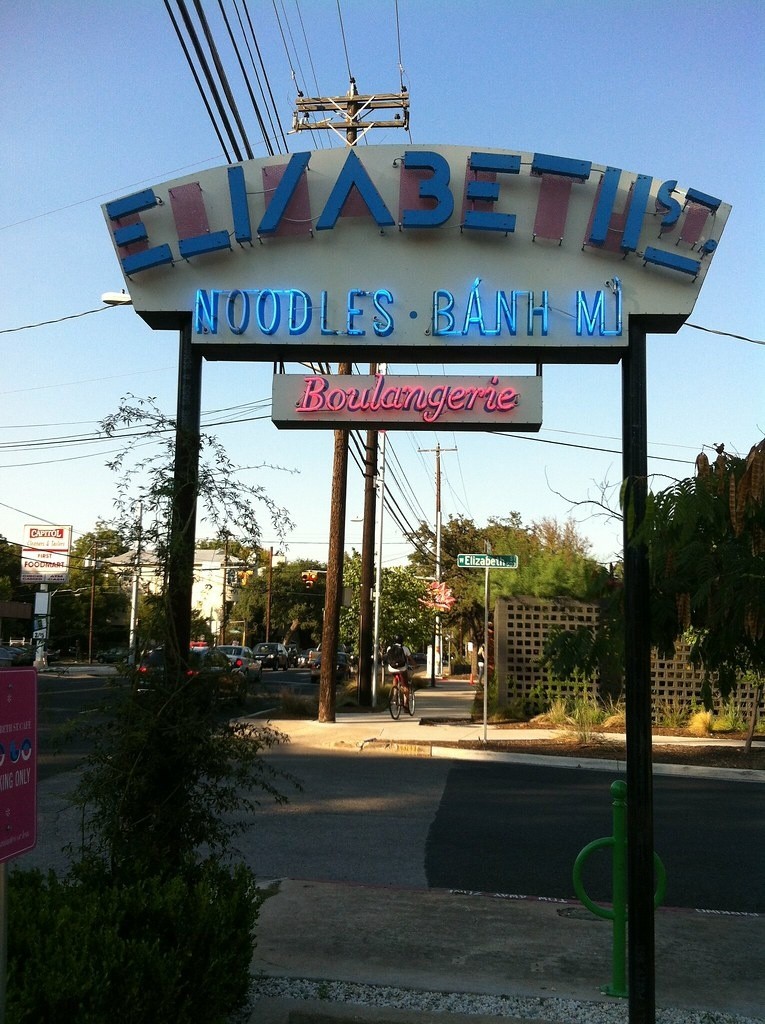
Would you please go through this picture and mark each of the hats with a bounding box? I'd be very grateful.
[394,635,403,643]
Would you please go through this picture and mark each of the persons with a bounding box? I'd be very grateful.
[387,635,417,710]
[478,646,485,684]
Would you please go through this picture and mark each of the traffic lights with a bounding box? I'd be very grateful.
[302,572,318,589]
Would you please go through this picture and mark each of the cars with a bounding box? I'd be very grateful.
[133,640,352,705]
[0,646,56,665]
[96,647,133,664]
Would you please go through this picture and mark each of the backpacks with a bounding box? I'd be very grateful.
[385,645,407,669]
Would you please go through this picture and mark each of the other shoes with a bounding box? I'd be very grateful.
[404,703,408,708]
[390,698,397,702]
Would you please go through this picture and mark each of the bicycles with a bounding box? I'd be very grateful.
[388,665,421,721]
